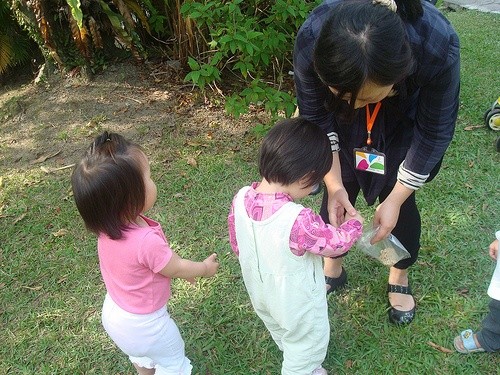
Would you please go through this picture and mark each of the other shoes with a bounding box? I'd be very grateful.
[311,366,327,375]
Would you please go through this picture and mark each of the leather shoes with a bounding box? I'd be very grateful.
[386,284,416,326]
[324,266,347,295]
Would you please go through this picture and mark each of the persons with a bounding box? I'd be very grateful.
[455,231,500,354]
[71,130,219,375]
[227,116,366,375]
[293,0,460,329]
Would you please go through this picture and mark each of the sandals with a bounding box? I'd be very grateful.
[454,329,486,353]
